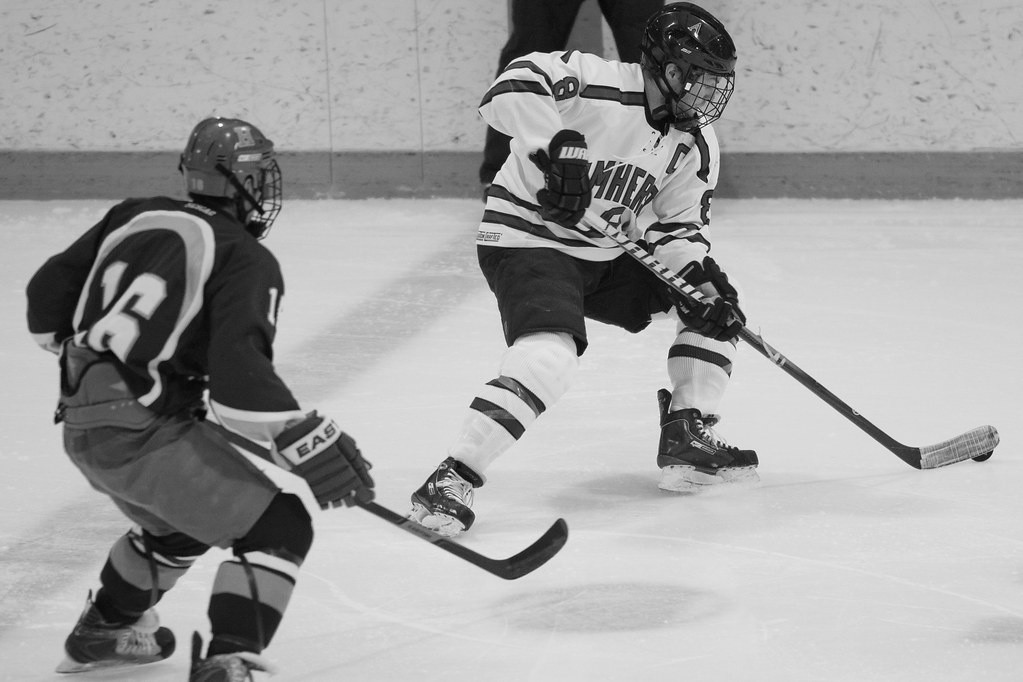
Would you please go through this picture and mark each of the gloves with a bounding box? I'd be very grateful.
[535,130,591,228]
[669,256,746,340]
[271,409,375,512]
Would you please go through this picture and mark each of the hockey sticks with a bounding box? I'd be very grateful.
[582,207,1001,471]
[203,416,571,581]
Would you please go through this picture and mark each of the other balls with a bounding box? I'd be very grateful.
[972,450,993,462]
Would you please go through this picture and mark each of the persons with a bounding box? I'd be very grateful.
[26,115,376,681]
[406,1,758,533]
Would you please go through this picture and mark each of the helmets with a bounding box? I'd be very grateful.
[639,1,738,133]
[181,117,280,239]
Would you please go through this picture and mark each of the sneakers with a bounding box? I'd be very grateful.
[189,631,271,682]
[407,456,483,539]
[656,388,759,492]
[55,588,176,675]
